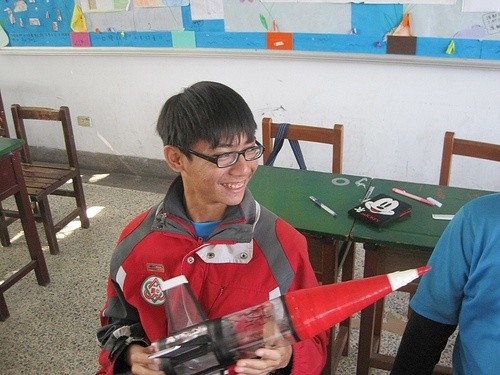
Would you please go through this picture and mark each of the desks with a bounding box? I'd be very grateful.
[0,134,51,322]
[247,165,372,375]
[349,178,500,375]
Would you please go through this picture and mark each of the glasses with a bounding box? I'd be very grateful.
[174,140,265,168]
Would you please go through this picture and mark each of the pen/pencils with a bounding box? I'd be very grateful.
[391,187,435,207]
[308,195,338,217]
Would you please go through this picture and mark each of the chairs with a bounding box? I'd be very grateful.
[0,104,89,255]
[439,131,500,186]
[262,118,344,174]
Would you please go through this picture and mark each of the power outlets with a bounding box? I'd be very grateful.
[78,115,91,127]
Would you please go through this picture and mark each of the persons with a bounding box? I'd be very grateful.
[391,194,500,375]
[96,81,331,375]
